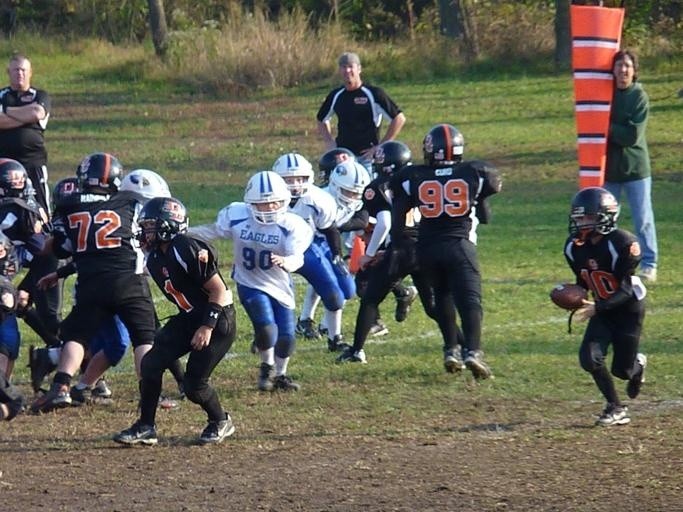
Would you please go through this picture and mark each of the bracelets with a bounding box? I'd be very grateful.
[200,300,223,330]
[1,104,7,114]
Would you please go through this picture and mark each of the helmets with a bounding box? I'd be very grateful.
[328,158,373,210]
[117,168,173,203]
[372,141,413,176]
[423,122,466,166]
[568,187,621,235]
[0,152,122,205]
[132,195,189,247]
[274,153,315,198]
[319,146,356,183]
[244,169,290,225]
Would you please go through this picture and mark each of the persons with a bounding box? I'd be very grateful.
[0,53,56,222]
[187,169,313,392]
[557,186,653,428]
[312,51,406,260]
[109,195,241,450]
[601,49,660,286]
[0,153,188,422]
[251,142,419,363]
[387,123,505,382]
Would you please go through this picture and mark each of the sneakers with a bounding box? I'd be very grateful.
[201,412,236,444]
[29,345,114,413]
[294,316,388,366]
[113,419,158,445]
[627,351,647,398]
[257,361,274,391]
[158,397,180,409]
[177,372,188,401]
[640,266,657,280]
[596,403,632,425]
[461,347,491,380]
[394,284,418,322]
[273,375,300,392]
[442,347,466,372]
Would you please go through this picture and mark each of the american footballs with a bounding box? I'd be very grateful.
[550,284,589,310]
